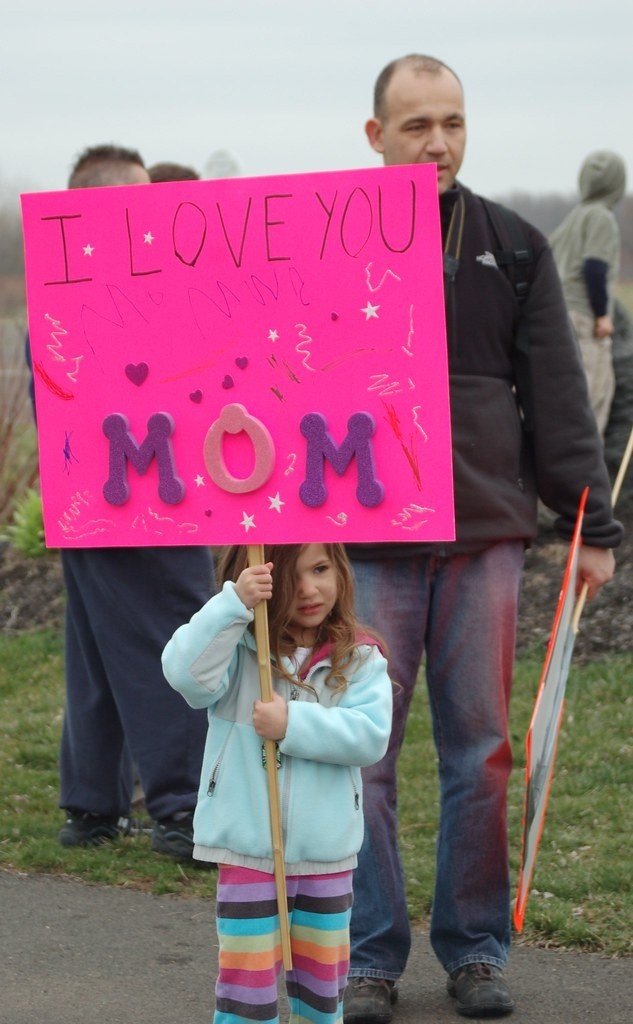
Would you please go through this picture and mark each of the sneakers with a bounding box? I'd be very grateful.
[150,809,214,870]
[341,974,400,1023]
[58,805,150,850]
[448,958,515,1016]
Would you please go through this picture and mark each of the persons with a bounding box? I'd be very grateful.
[162,543,394,1024]
[313,55,622,1024]
[26,145,220,873]
[547,152,627,453]
